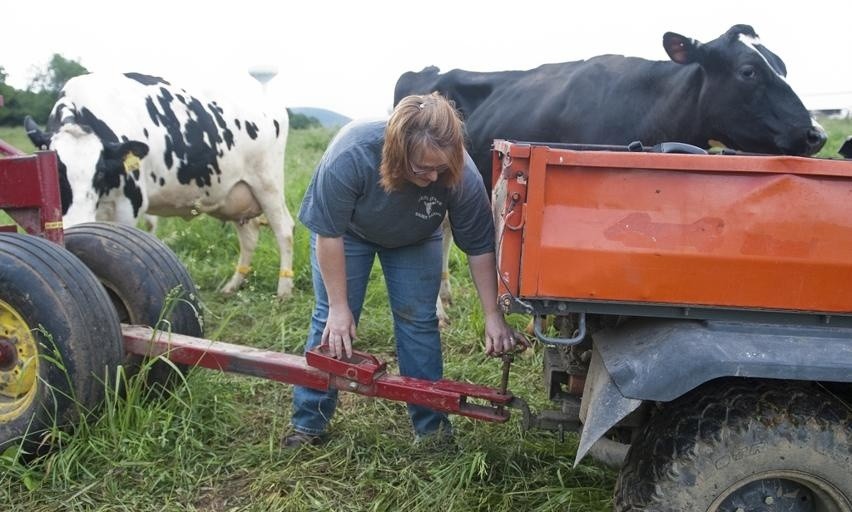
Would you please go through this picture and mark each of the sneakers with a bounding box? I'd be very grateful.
[282,433,322,451]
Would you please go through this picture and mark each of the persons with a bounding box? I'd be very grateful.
[278,89,533,462]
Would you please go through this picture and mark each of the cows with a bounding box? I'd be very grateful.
[392,22,828,333]
[22,69,297,307]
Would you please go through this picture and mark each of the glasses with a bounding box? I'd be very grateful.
[409,160,448,176]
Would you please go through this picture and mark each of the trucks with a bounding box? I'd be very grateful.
[490,136,851,511]
